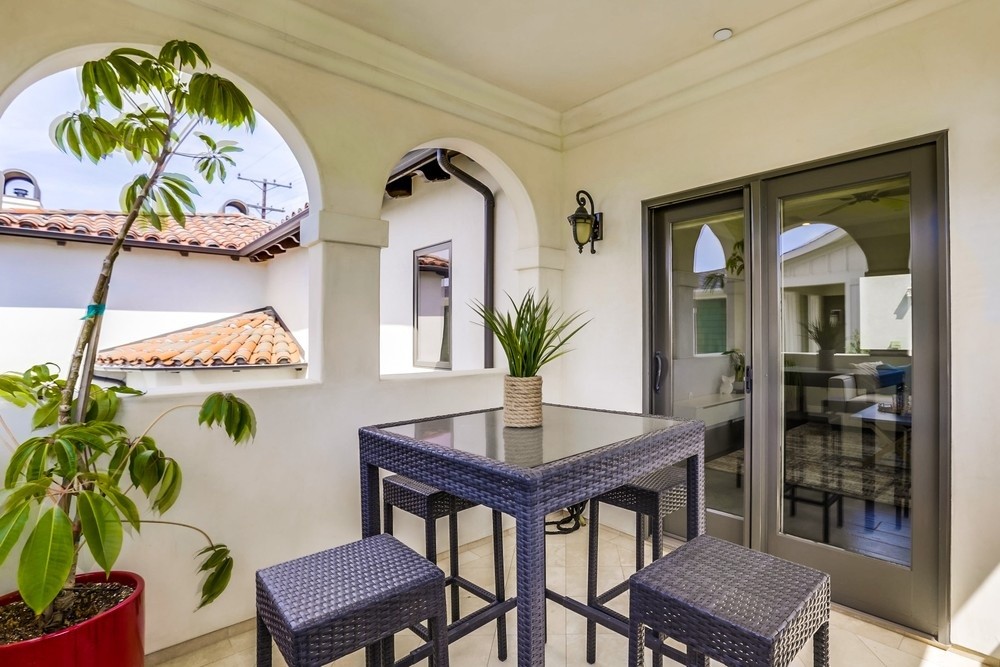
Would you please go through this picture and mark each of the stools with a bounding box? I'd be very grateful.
[628,535,832,667]
[586,468,687,667]
[255,532,449,667]
[383,474,507,667]
[786,398,912,544]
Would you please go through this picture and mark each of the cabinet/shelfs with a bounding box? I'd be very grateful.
[680,393,744,463]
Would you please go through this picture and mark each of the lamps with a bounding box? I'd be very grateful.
[567,190,603,254]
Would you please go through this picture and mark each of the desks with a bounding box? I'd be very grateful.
[359,396,706,667]
[784,365,906,530]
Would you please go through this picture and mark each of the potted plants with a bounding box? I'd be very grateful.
[798,310,846,372]
[721,349,745,391]
[463,289,595,427]
[0,362,257,667]
